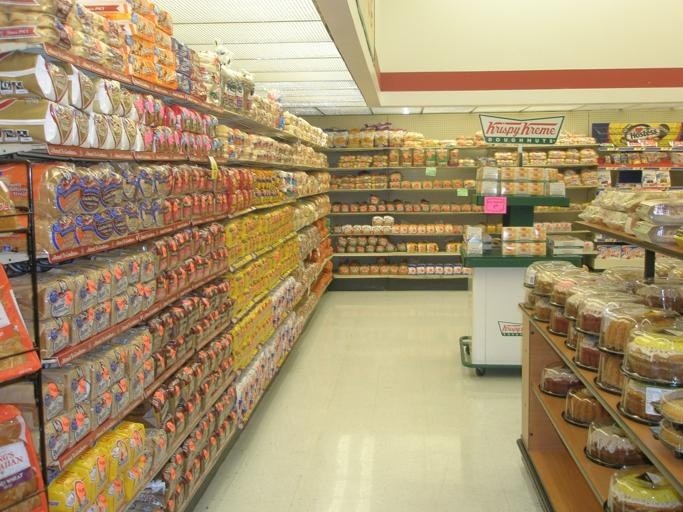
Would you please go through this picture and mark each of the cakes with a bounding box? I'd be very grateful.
[527,271,683,512]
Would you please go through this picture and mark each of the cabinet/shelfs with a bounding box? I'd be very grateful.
[0,1,330,512]
[329,141,603,292]
[599,144,682,192]
[516,203,683,510]
[588,236,644,269]
